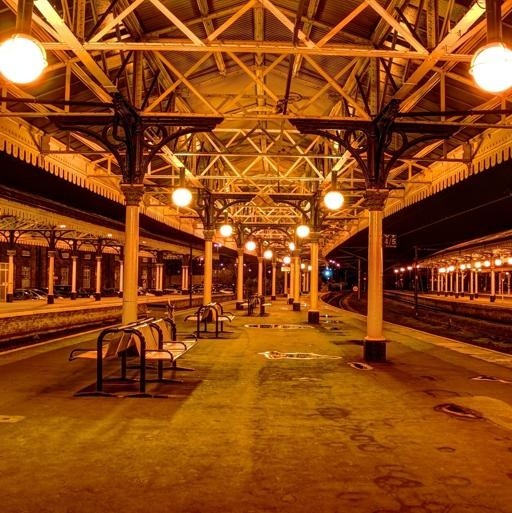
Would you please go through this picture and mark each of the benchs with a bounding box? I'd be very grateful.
[68,293,273,399]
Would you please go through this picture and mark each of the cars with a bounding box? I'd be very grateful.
[14,281,236,299]
